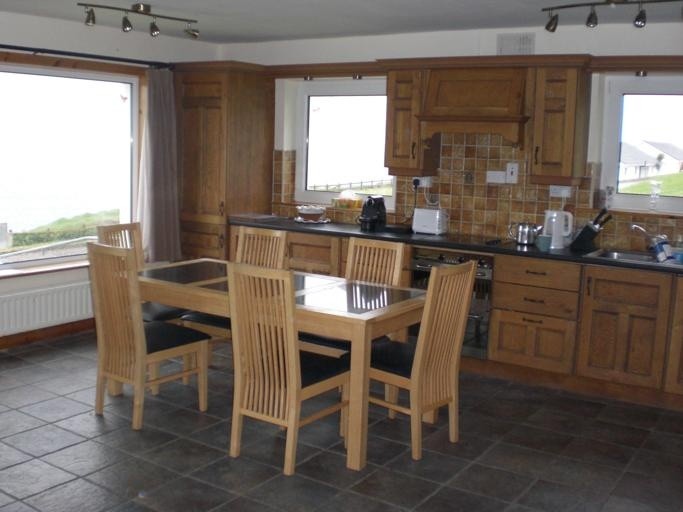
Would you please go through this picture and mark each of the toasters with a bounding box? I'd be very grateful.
[411,206,451,236]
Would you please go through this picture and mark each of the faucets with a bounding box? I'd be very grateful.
[629,223,650,238]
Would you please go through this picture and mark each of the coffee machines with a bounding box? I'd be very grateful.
[358,195,387,233]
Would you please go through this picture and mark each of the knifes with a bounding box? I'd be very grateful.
[592,208,612,227]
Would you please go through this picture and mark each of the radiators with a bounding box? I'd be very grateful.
[0,279,95,340]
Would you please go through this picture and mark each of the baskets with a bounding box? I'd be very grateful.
[325,207,362,224]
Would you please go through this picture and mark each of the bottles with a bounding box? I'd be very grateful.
[648,179,661,210]
[671,235,683,265]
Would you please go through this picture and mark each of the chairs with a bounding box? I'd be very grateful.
[341,237,412,402]
[179,226,289,385]
[96,222,195,397]
[226,262,349,476]
[87,242,209,431]
[335,259,478,462]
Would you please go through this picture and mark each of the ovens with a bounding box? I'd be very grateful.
[400,259,492,362]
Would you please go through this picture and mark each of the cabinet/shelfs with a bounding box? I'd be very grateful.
[382,57,438,179]
[415,66,528,149]
[665,270,683,395]
[172,66,272,219]
[527,53,592,188]
[174,220,227,261]
[487,250,582,376]
[343,233,410,341]
[229,224,341,275]
[580,260,675,391]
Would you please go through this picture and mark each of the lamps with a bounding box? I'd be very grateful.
[541,1,651,33]
[77,2,200,41]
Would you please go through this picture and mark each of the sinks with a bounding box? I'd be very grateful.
[582,246,653,265]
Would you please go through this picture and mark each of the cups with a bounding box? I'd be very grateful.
[534,234,552,252]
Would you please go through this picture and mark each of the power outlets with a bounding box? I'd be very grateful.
[410,176,432,189]
[547,184,572,200]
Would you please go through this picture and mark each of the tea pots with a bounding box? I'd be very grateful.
[505,221,543,244]
[540,209,572,248]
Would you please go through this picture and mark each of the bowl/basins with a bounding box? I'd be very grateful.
[295,206,327,221]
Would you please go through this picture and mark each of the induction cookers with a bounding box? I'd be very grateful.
[403,233,506,247]
